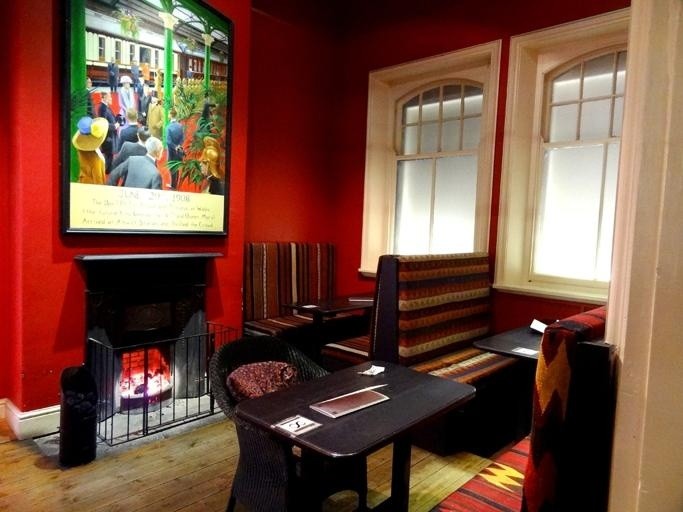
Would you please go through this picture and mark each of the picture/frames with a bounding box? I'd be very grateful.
[49,0,236,239]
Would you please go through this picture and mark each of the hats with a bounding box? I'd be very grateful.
[119,76,133,84]
[152,90,157,97]
[72,117,109,151]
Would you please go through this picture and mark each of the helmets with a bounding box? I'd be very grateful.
[198,136,225,179]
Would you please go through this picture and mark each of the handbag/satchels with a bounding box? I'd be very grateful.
[226,362,301,402]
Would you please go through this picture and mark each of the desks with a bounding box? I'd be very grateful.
[469,319,559,458]
[229,354,479,510]
[274,291,371,359]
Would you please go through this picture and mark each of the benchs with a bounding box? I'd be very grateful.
[314,253,396,372]
[431,304,607,510]
[233,236,369,373]
[373,247,529,459]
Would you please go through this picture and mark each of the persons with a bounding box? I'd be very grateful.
[72,57,226,199]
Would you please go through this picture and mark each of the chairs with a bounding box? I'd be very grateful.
[208,333,353,510]
